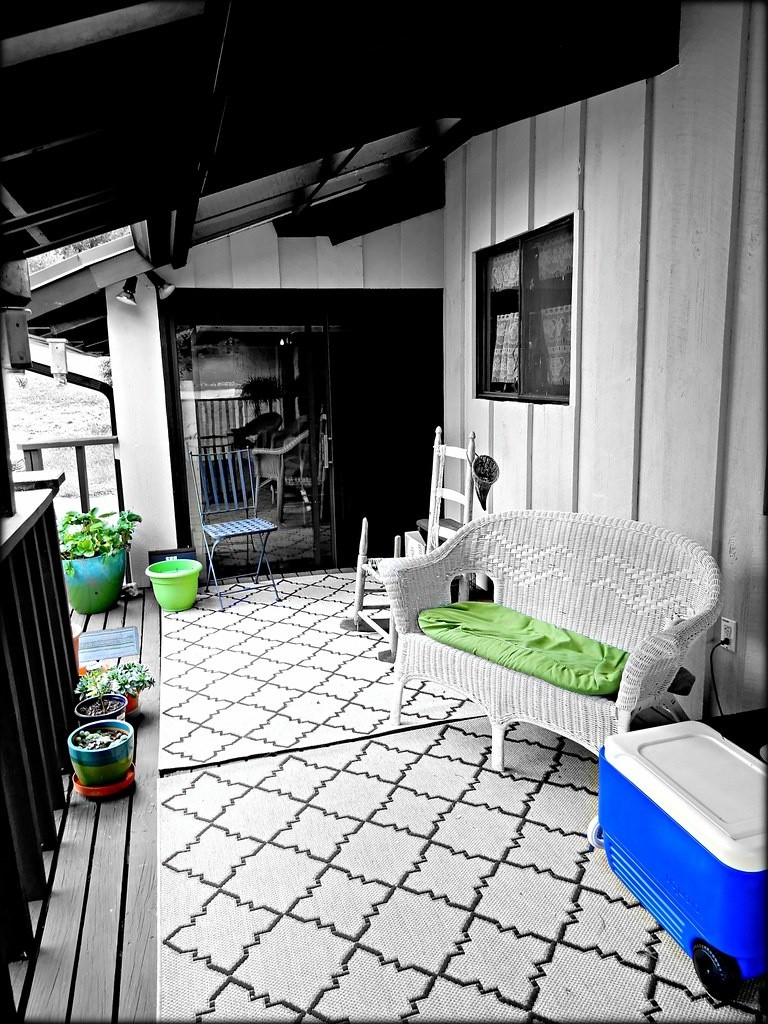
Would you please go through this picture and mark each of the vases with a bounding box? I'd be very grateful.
[68,719,135,796]
[144,559,203,612]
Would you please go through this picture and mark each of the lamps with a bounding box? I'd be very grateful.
[116,276,138,305]
[146,271,176,299]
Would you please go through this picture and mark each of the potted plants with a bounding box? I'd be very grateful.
[57,508,142,615]
[74,672,128,725]
[75,663,156,714]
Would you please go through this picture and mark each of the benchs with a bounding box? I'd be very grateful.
[376,509,721,848]
[252,414,327,524]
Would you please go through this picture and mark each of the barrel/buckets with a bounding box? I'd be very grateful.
[145,559,203,612]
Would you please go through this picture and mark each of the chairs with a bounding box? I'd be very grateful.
[354,426,476,659]
[231,412,283,481]
[189,446,282,612]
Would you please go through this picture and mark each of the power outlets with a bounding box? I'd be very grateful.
[720,617,738,653]
